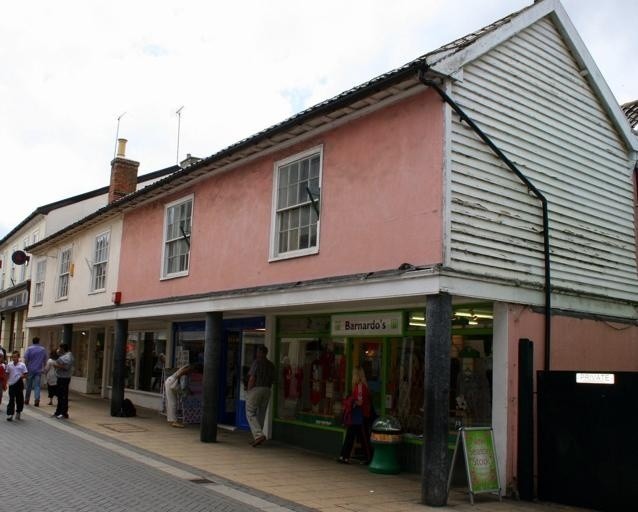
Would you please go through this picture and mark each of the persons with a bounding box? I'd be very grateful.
[245,346,275,447]
[0,336,73,421]
[164,360,201,427]
[334,366,377,465]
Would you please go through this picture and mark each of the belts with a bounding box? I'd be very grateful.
[254,384,270,387]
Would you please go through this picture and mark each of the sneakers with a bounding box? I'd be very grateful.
[252,435,266,448]
[34,400,39,407]
[25,399,29,405]
[7,415,13,421]
[360,459,375,465]
[336,455,352,465]
[170,421,185,429]
[15,413,21,420]
[56,413,69,419]
[48,402,53,405]
[51,412,63,418]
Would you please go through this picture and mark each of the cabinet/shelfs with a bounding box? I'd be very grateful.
[160,367,203,424]
